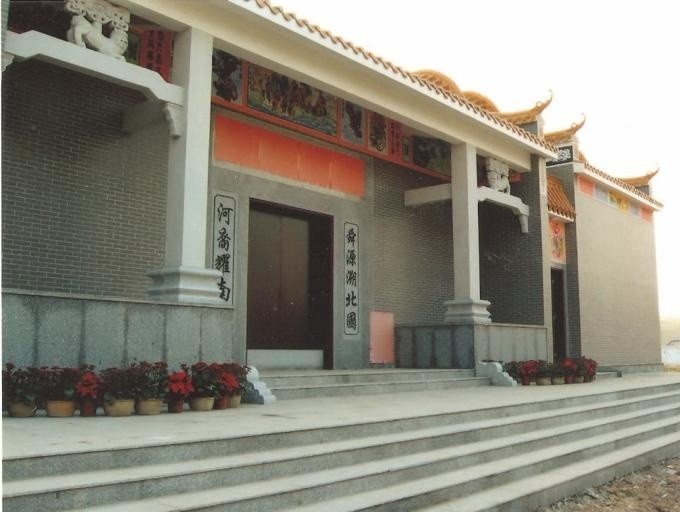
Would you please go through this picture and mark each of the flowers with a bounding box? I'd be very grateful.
[2,360,254,401]
[502,353,599,376]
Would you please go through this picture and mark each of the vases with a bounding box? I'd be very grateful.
[7,394,245,415]
[513,374,594,384]
